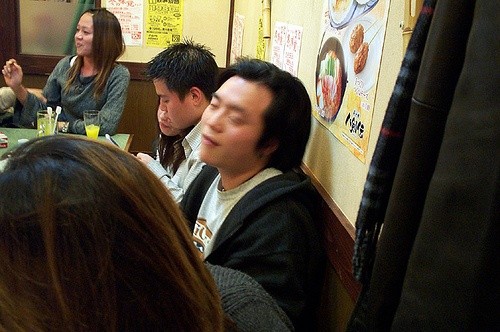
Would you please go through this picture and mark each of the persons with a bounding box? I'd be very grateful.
[143,42,220,203]
[2,8,131,136]
[180,58,323,332]
[0,134,295,332]
[153,98,184,177]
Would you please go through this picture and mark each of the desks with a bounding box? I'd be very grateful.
[0,126,134,161]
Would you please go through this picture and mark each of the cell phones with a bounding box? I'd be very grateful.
[105,133,119,148]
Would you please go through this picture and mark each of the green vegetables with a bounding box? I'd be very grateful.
[326,56,335,77]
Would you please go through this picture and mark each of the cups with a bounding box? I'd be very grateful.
[37,110,57,137]
[83,110,101,139]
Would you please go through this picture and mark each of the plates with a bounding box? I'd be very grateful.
[343,19,383,96]
[328,0,357,28]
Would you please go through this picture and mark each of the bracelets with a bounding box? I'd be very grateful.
[62,121,69,132]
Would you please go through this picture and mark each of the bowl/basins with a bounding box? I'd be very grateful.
[315,37,346,124]
